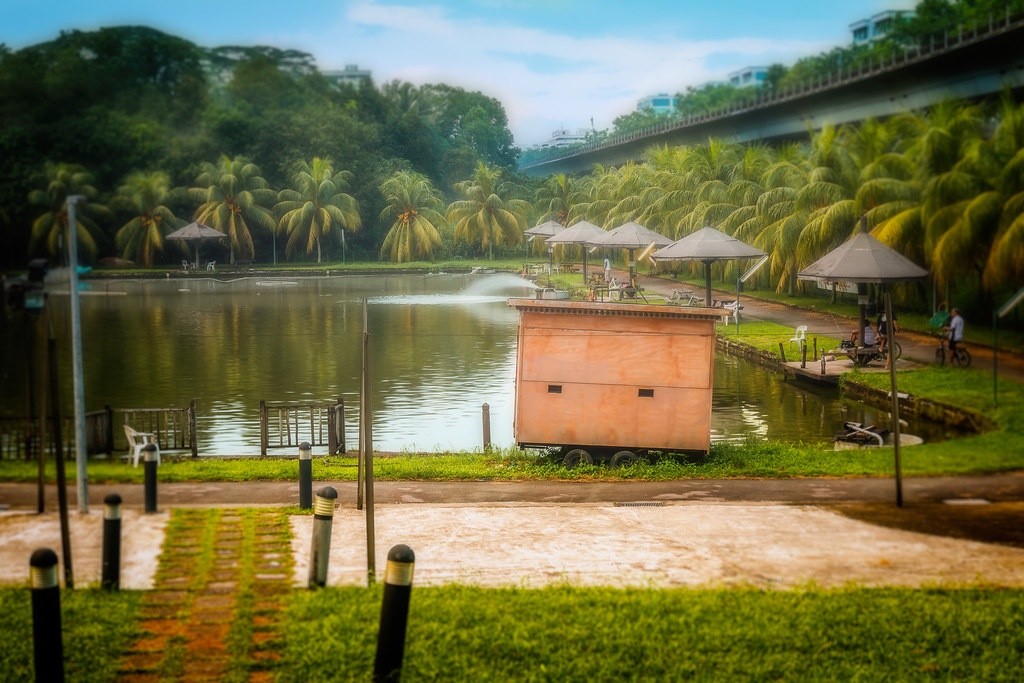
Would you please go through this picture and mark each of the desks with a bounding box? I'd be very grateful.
[671,287,693,304]
[712,296,736,308]
[560,264,573,273]
[588,271,605,284]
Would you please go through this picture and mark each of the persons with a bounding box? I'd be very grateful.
[851,320,875,347]
[523,265,528,278]
[943,309,964,364]
[877,312,898,370]
[603,256,611,282]
[625,275,636,297]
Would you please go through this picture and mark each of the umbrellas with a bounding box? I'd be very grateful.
[649,221,766,306]
[545,220,608,283]
[585,222,674,279]
[522,220,567,266]
[166,221,229,266]
[796,217,929,347]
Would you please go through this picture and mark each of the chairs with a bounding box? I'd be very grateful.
[609,284,621,300]
[123,425,162,469]
[722,301,741,326]
[789,325,808,354]
[608,278,617,289]
[681,296,695,307]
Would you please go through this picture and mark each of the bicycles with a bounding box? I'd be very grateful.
[875,335,902,362]
[936,330,971,369]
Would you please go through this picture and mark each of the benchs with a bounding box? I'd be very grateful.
[571,268,579,272]
[689,294,704,302]
[587,277,601,282]
[738,306,744,310]
